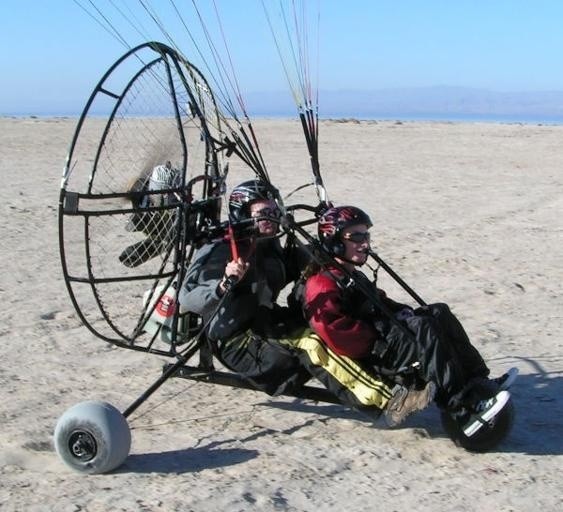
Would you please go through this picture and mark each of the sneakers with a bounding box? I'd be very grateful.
[485,367,519,397]
[461,390,512,437]
[384,381,437,428]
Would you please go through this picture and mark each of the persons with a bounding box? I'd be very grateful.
[301,204,519,439]
[178,179,435,429]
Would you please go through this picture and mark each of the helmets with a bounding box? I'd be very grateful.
[229,179,282,227]
[316,205,373,246]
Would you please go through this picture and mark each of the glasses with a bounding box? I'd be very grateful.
[341,232,371,243]
[247,207,283,219]
